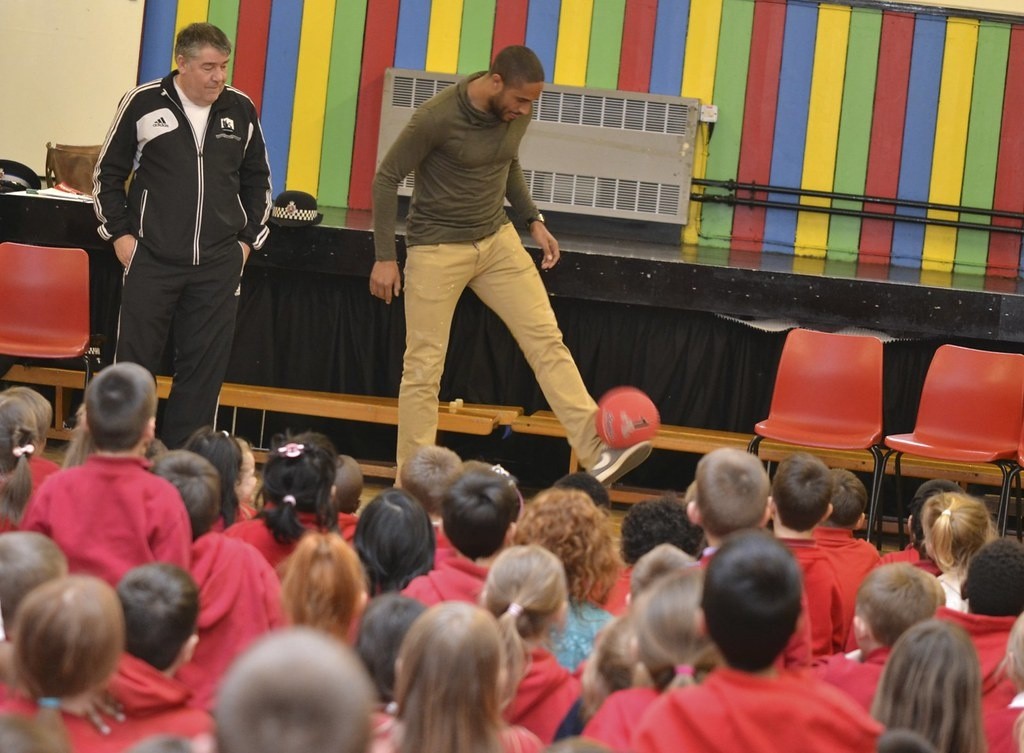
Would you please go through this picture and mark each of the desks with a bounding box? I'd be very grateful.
[0,186,1024,521]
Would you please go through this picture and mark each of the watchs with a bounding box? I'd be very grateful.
[525,214,546,227]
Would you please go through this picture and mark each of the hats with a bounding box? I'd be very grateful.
[267,189,323,227]
[0,159,42,193]
[912,477,965,502]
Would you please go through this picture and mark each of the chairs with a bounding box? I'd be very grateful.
[746,328,886,542]
[872,344,1024,552]
[0,237,107,393]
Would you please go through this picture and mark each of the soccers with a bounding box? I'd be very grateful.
[595,386,661,449]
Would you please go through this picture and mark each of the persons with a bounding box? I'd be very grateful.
[92,23,272,450]
[0,364,1024,753]
[369,45,651,493]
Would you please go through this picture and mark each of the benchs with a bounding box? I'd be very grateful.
[511,410,759,508]
[152,373,525,480]
[752,437,1024,538]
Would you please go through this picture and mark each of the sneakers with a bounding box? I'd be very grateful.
[588,439,653,485]
